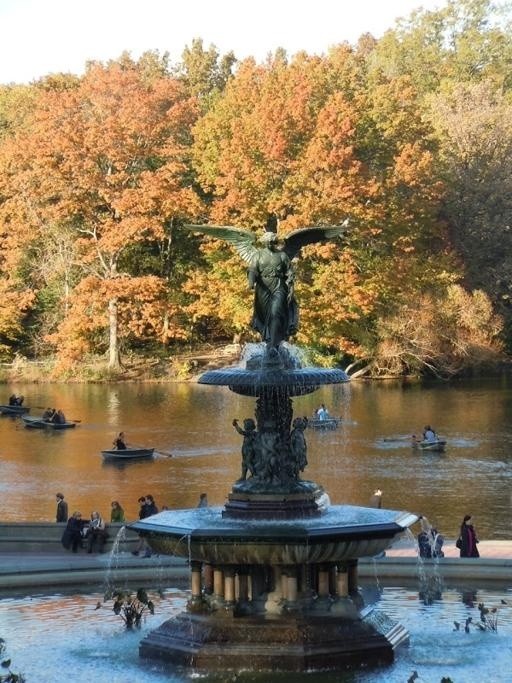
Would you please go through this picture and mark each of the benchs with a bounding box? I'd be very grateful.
[0,522,139,552]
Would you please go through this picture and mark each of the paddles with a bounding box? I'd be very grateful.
[126,443,171,457]
[65,419,81,423]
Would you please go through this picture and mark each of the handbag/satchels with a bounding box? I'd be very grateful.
[455,537,463,548]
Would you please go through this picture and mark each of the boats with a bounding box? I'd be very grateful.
[308,419,337,430]
[0,406,30,415]
[21,416,75,430]
[101,448,154,462]
[412,439,447,451]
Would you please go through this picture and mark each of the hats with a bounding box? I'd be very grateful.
[56,493,64,499]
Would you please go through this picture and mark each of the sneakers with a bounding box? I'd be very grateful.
[131,550,151,558]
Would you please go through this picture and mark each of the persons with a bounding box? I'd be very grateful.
[110,502,125,522]
[313,404,328,420]
[56,493,68,523]
[10,396,24,406]
[232,418,256,481]
[249,232,299,348]
[459,516,479,558]
[132,495,158,558]
[62,511,109,553]
[409,424,435,441]
[113,432,126,450]
[43,408,65,424]
[418,523,444,558]
[290,417,308,479]
